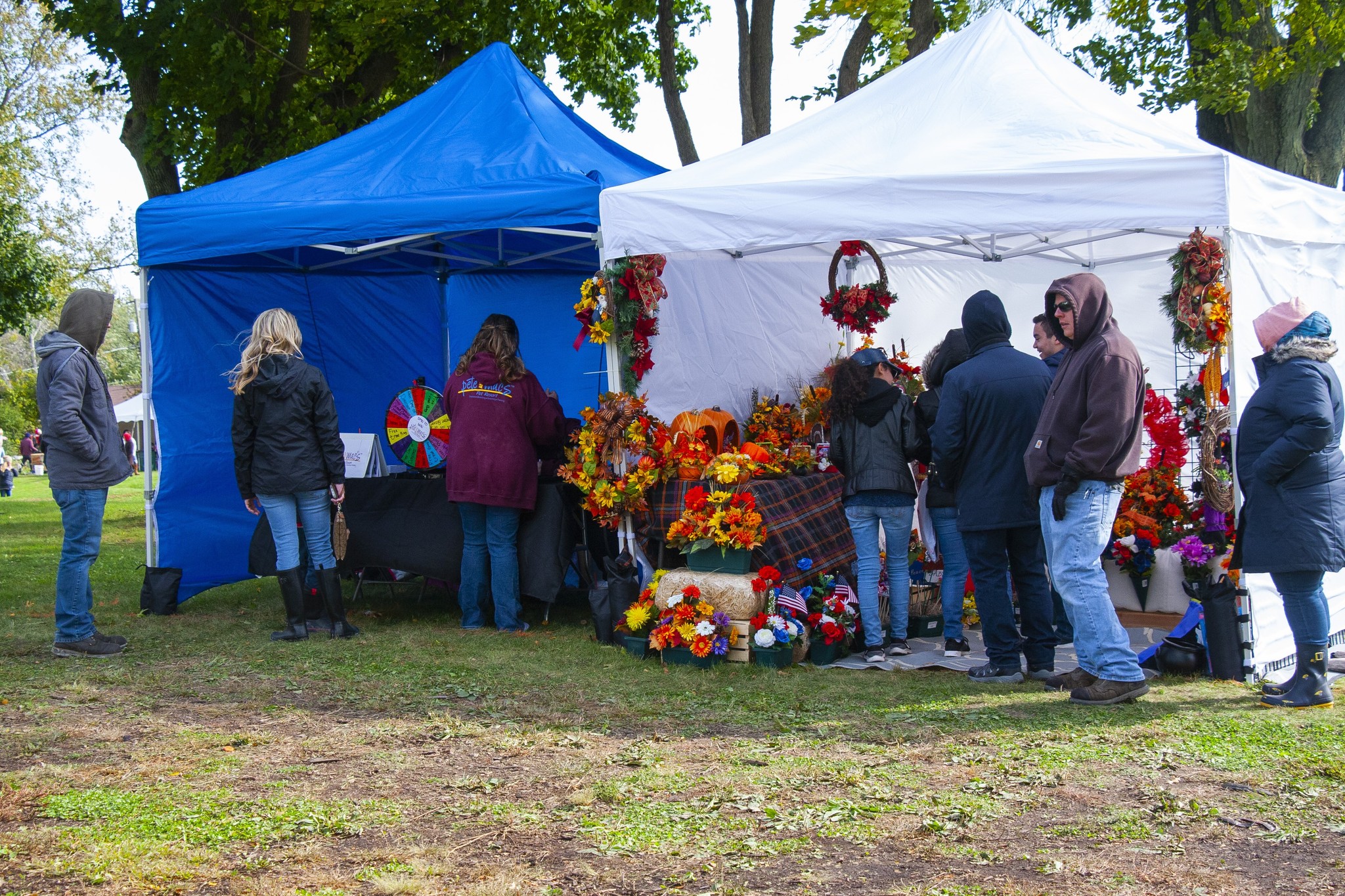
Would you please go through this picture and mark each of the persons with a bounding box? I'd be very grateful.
[37,288,134,658]
[1023,274,1151,706]
[219,308,360,644]
[828,348,923,663]
[0,427,159,497]
[914,328,1013,657]
[1227,295,1345,711]
[930,288,1055,685]
[1033,313,1068,379]
[536,417,581,475]
[444,313,564,633]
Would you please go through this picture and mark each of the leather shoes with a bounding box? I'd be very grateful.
[51,635,123,658]
[1070,678,1149,705]
[92,631,128,649]
[1043,668,1099,691]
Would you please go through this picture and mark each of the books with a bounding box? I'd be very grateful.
[339,432,389,479]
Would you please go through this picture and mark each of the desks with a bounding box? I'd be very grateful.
[248,476,595,625]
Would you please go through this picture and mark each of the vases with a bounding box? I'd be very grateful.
[751,647,793,670]
[661,648,726,668]
[624,636,656,659]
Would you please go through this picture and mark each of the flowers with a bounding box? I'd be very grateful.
[558,230,1241,658]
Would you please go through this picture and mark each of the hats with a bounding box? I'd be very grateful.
[849,348,903,378]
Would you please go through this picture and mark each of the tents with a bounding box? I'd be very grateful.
[136,37,672,613]
[112,392,154,471]
[596,2,1345,682]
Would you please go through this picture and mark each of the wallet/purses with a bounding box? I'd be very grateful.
[332,483,350,561]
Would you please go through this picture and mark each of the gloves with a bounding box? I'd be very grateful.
[1051,461,1086,521]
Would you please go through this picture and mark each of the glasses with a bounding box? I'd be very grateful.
[881,363,898,378]
[1053,301,1074,313]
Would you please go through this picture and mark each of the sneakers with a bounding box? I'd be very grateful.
[1055,629,1074,648]
[889,638,912,656]
[967,662,1025,684]
[1026,664,1055,679]
[944,636,972,657]
[864,645,886,662]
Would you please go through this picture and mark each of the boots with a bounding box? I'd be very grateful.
[269,565,309,643]
[1262,666,1297,695]
[314,563,360,638]
[1260,643,1334,710]
[302,582,332,632]
